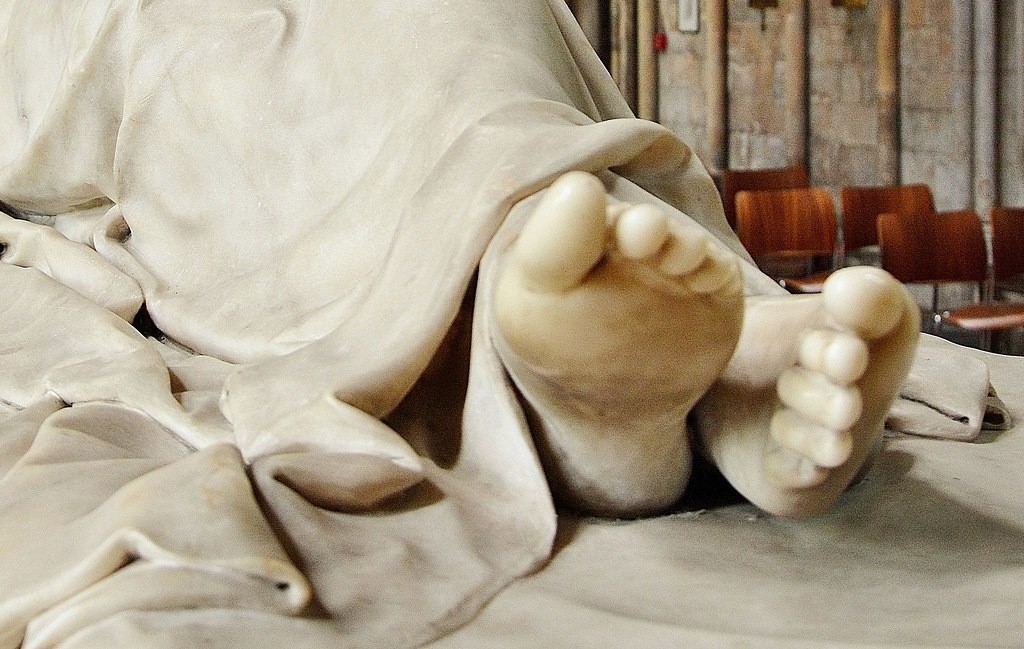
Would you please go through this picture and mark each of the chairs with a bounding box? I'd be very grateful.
[719,167,1024,353]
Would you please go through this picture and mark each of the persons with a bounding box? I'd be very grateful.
[492,168,924,520]
